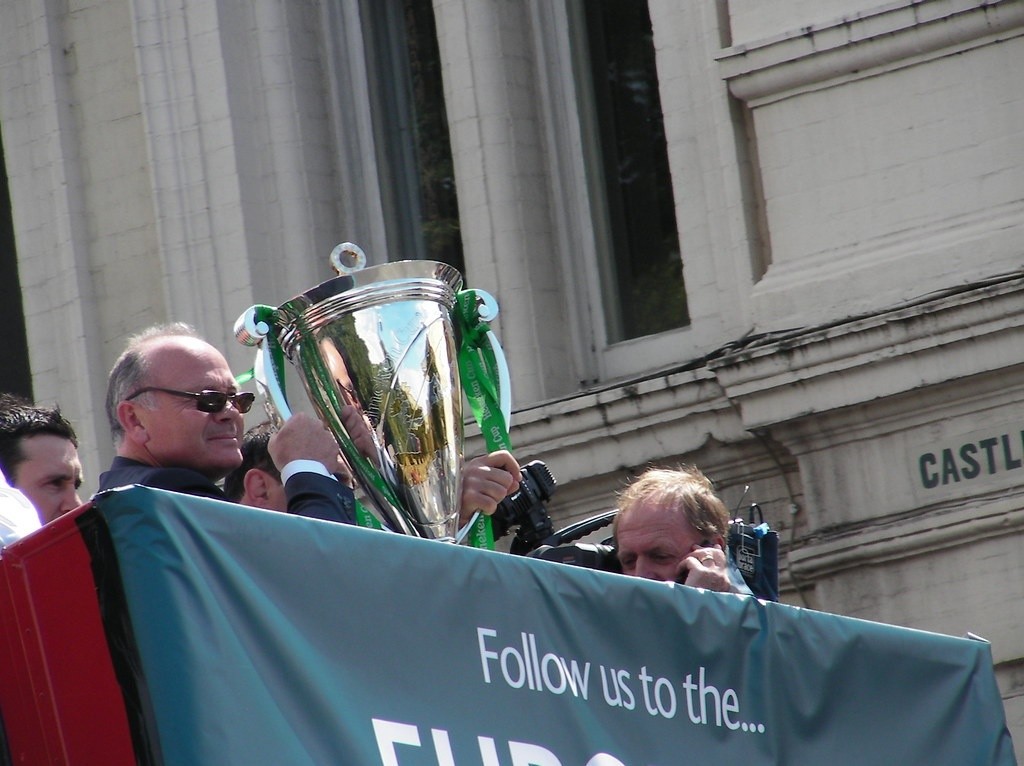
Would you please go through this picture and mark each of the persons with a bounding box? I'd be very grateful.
[224,421,290,512]
[94,320,357,527]
[0,394,84,528]
[460,451,731,594]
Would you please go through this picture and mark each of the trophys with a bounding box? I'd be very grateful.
[234,242,514,547]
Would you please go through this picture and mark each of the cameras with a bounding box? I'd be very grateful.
[491,460,558,557]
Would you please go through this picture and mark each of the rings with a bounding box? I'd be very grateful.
[701,555,717,565]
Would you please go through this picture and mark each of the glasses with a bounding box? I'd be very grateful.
[122,384,254,413]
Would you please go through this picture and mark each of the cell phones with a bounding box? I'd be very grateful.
[675,539,715,585]
